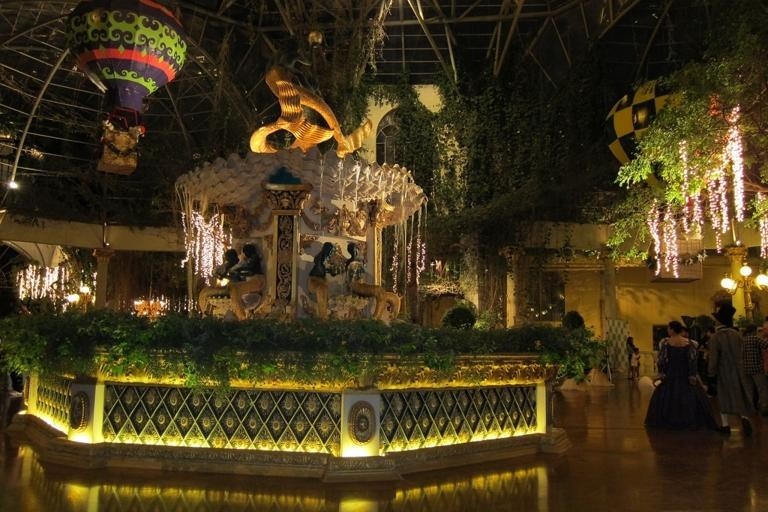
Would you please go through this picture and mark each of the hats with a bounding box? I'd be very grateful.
[711,303,737,328]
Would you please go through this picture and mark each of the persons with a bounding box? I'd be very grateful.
[211,248,240,278]
[623,300,768,439]
[345,241,366,278]
[236,243,261,276]
[309,241,337,278]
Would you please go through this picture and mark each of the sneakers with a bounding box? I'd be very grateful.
[719,417,754,437]
